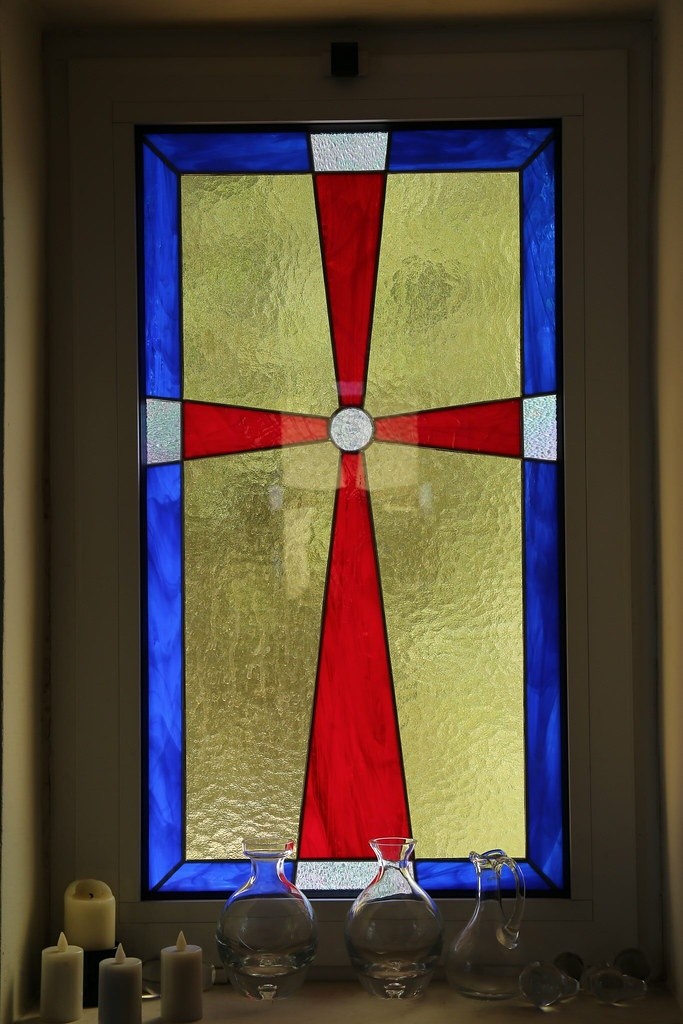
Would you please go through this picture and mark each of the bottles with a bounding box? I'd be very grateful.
[343,836,441,1000]
[216,847,317,998]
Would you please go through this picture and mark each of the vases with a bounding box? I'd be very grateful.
[214,835,316,1002]
[343,836,444,1003]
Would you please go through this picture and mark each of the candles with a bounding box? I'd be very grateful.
[39,932,83,1023]
[98,943,142,1024]
[63,879,116,950]
[161,931,203,1023]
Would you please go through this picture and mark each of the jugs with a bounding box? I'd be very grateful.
[445,849,525,1001]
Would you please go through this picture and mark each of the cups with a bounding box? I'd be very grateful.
[519,958,648,1013]
